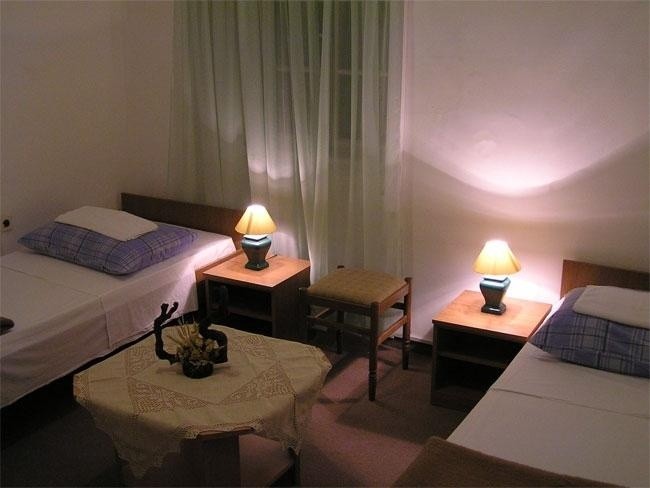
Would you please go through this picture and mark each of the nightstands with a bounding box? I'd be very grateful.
[430,289,553,414]
[202,252,310,338]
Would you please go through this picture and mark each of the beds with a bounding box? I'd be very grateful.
[0,192,245,408]
[389,259,649,487]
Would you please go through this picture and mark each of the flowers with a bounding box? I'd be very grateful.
[166,313,225,361]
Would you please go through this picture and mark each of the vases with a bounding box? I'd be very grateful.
[181,361,213,380]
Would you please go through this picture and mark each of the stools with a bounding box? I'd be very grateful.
[299,265,413,401]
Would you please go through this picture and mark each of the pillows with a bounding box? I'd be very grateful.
[527,287,650,380]
[16,221,199,276]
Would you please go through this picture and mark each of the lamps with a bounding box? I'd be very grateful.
[472,239,522,316]
[234,204,277,272]
